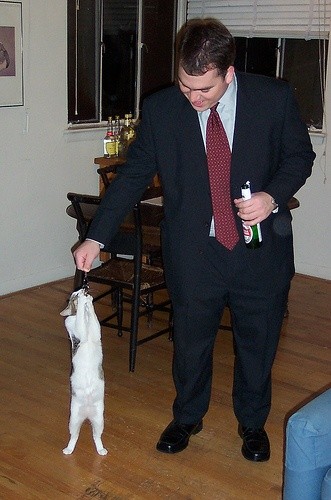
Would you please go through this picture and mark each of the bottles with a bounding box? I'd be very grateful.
[240,184,263,249]
[104,114,136,158]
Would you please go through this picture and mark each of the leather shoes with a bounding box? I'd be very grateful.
[156,418,204,454]
[238,423,270,462]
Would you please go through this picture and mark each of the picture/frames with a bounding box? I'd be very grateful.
[0,1,24,107]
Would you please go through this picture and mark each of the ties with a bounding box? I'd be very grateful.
[206,102,240,251]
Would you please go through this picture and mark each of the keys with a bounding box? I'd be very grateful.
[83,270,89,291]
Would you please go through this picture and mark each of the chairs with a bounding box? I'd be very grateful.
[66,192,173,374]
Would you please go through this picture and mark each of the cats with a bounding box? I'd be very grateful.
[60,288,109,456]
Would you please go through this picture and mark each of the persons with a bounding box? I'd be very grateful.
[72,17,315,462]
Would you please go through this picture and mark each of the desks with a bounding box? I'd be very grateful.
[95,157,160,264]
[66,186,163,324]
